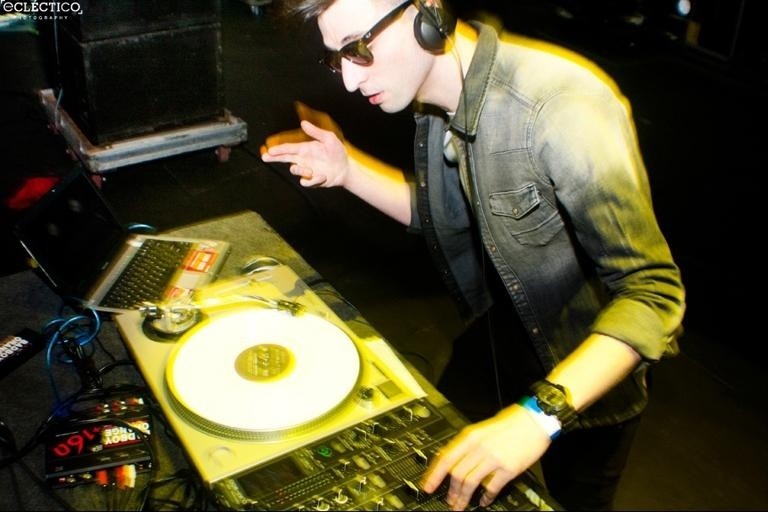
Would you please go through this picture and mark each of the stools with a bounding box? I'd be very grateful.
[37,87,248,190]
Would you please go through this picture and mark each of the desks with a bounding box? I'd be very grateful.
[0,210,565,512]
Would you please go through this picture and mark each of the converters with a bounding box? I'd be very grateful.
[0,327,47,381]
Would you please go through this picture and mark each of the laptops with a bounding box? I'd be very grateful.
[11,166,232,313]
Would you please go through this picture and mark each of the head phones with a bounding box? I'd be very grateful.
[414,0,455,55]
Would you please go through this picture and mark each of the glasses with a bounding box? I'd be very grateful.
[315,0,417,77]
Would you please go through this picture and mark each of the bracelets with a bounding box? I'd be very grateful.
[517,395,561,442]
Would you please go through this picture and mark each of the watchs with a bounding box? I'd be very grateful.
[532,381,578,437]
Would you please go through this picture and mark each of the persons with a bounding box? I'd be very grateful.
[257,2,689,511]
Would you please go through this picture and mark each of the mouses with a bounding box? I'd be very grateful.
[240,255,283,277]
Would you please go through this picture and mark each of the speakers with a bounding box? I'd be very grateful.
[38,0,222,141]
